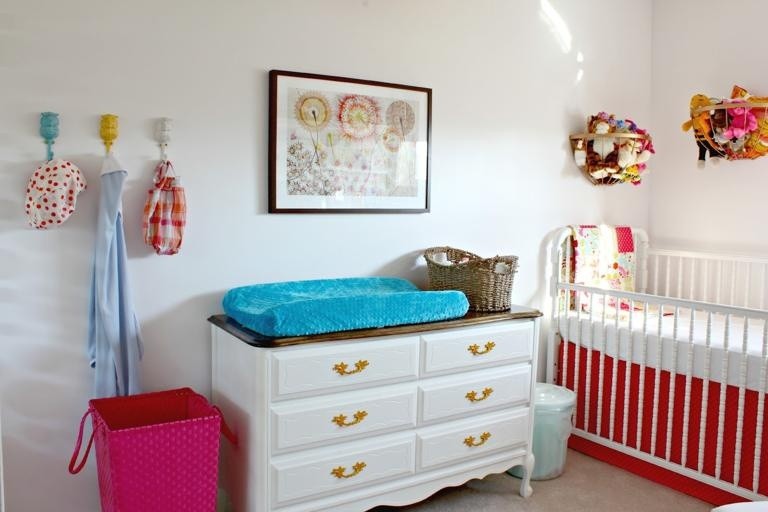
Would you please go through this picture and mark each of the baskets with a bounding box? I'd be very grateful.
[687,101,768,159]
[570,130,650,186]
[424,247,519,312]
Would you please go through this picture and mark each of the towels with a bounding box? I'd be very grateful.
[84,148,143,401]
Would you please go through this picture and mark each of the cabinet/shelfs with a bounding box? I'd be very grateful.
[207,304,542,512]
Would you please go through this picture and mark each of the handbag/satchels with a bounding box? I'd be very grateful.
[142,162,187,256]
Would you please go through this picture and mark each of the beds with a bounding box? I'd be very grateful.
[545,225,768,505]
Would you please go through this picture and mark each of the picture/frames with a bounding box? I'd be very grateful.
[269,70,433,214]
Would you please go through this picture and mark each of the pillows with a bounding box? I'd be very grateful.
[223,277,471,339]
